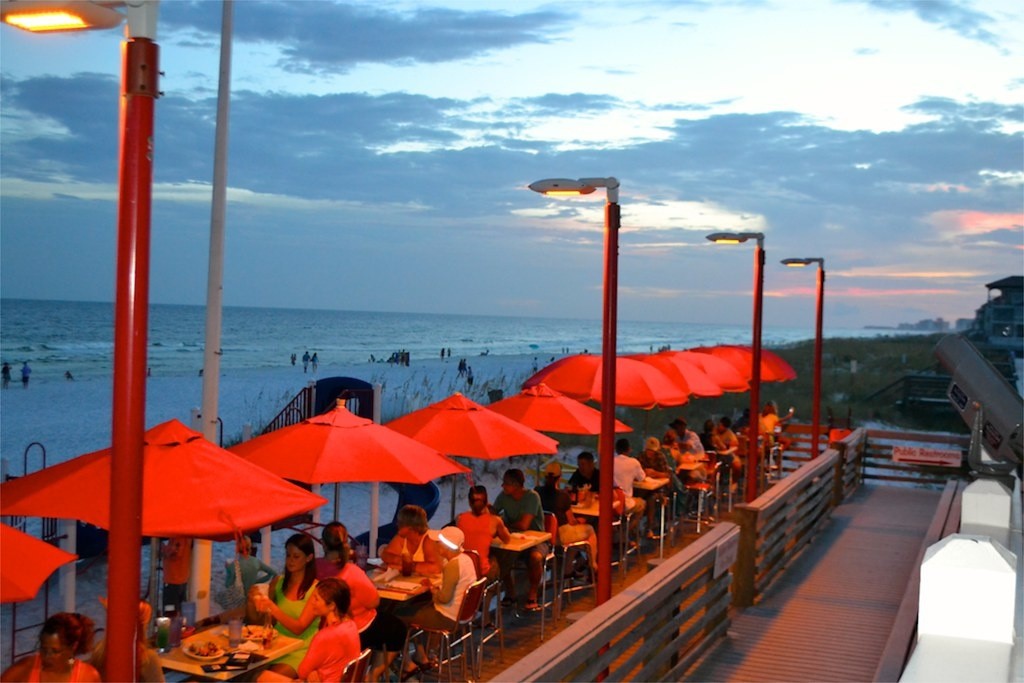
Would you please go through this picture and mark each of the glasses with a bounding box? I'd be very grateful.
[503,478,515,486]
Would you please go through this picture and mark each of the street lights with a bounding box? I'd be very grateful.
[705,231,766,501]
[0,1,166,683]
[528,176,621,682]
[781,257,826,459]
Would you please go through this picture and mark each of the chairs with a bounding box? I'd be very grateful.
[342,439,783,683]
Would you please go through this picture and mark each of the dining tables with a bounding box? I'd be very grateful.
[640,475,669,488]
[369,568,440,600]
[151,624,304,679]
[574,496,600,515]
[494,528,551,550]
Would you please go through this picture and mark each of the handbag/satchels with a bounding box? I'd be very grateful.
[215,560,246,610]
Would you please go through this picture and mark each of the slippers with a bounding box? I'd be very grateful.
[399,658,433,683]
[575,560,587,572]
[525,597,538,610]
[501,596,517,607]
[479,621,495,629]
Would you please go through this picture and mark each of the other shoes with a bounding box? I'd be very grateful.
[629,530,654,548]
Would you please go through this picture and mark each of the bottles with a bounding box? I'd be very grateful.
[578,486,586,507]
[263,609,274,651]
[571,481,577,505]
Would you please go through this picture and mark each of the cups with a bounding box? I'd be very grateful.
[356,554,366,573]
[156,627,168,649]
[402,553,412,577]
[254,584,269,611]
[229,620,242,648]
[168,618,182,646]
[357,546,366,558]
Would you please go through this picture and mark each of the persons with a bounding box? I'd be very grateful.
[0,612,102,683]
[313,521,405,683]
[379,401,795,613]
[161,535,192,614]
[225,536,274,611]
[251,578,361,683]
[1,347,589,387]
[244,533,323,678]
[399,525,479,683]
[85,595,167,683]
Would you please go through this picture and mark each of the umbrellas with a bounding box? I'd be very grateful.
[522,354,691,437]
[717,345,785,382]
[0,522,79,603]
[382,391,559,521]
[224,397,472,522]
[738,345,797,382]
[621,354,724,398]
[659,350,751,393]
[0,418,329,636]
[484,382,634,488]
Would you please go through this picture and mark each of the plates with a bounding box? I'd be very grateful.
[182,642,224,661]
[223,625,279,642]
[182,625,195,639]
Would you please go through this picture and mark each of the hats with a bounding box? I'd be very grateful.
[645,436,661,452]
[668,416,688,428]
[546,462,562,477]
[428,527,465,553]
